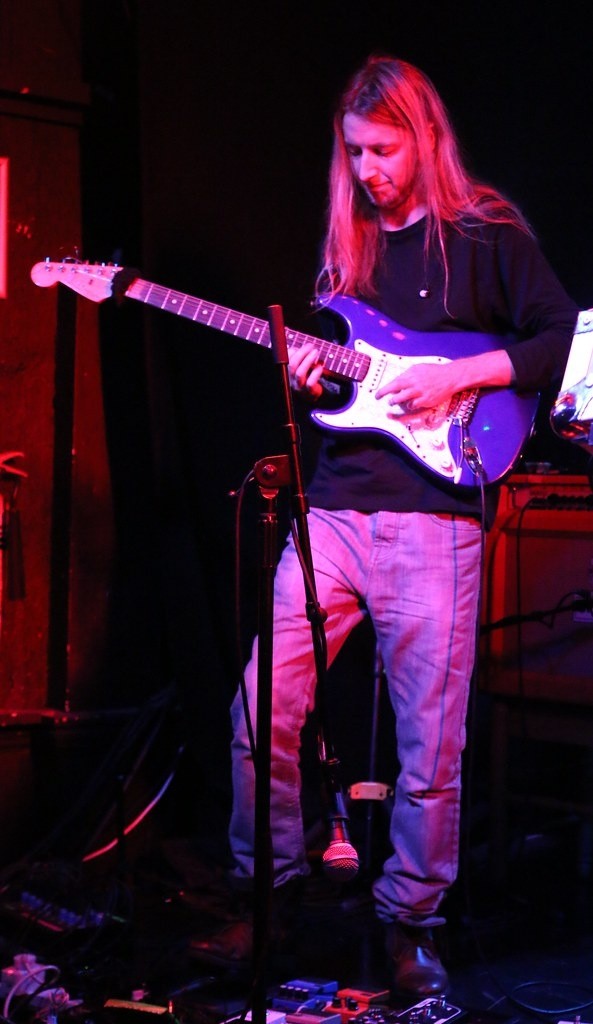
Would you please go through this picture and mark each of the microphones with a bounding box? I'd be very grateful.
[317,734,360,884]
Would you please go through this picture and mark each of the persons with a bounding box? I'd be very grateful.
[190,52,581,1002]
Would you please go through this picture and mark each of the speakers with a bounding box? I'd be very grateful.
[487,475,592,706]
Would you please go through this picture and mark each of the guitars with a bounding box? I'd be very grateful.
[30,256,544,501]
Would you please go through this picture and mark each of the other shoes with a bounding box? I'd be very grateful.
[382,916,451,1004]
[176,885,275,981]
